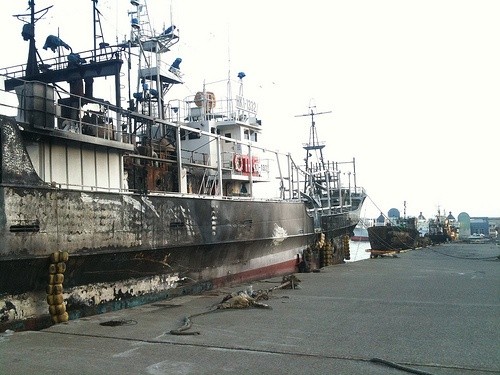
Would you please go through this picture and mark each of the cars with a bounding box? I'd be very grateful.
[466,231,490,245]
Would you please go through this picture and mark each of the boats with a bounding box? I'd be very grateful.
[366,201,457,259]
[0,0,366,332]
